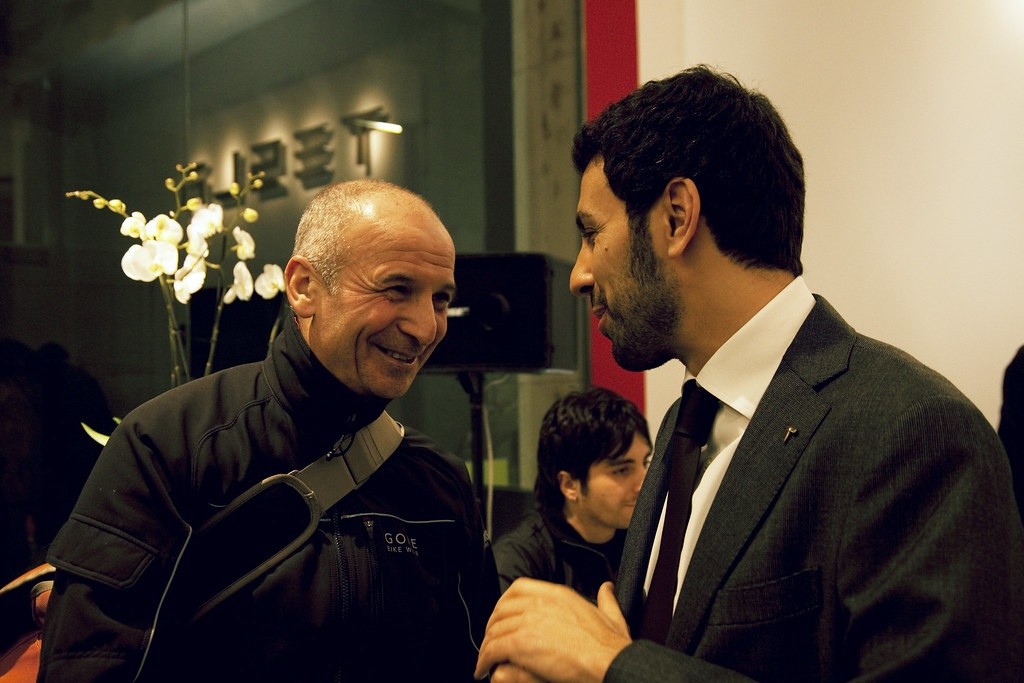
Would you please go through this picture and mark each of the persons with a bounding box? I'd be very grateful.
[0,550,56,683]
[473,64,1024,683]
[489,385,654,607]
[33,179,503,683]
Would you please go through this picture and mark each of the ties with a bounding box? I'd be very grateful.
[640,379,723,641]
